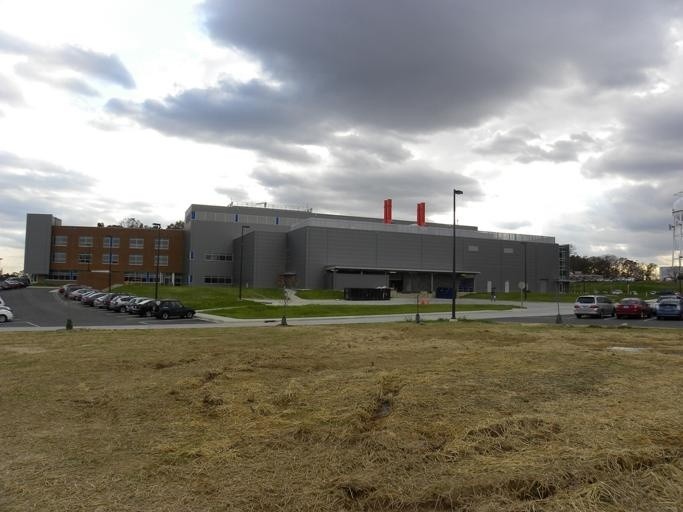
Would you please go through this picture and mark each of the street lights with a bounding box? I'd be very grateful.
[239,226,250,299]
[451,189,462,319]
[105,234,113,291]
[152,223,161,299]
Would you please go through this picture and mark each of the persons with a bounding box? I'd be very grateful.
[491,287,497,302]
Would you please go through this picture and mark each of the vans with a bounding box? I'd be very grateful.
[575,295,615,319]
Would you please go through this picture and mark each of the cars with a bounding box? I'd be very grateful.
[0,297,13,323]
[615,298,652,320]
[655,296,683,319]
[60,283,195,319]
[0,275,30,289]
